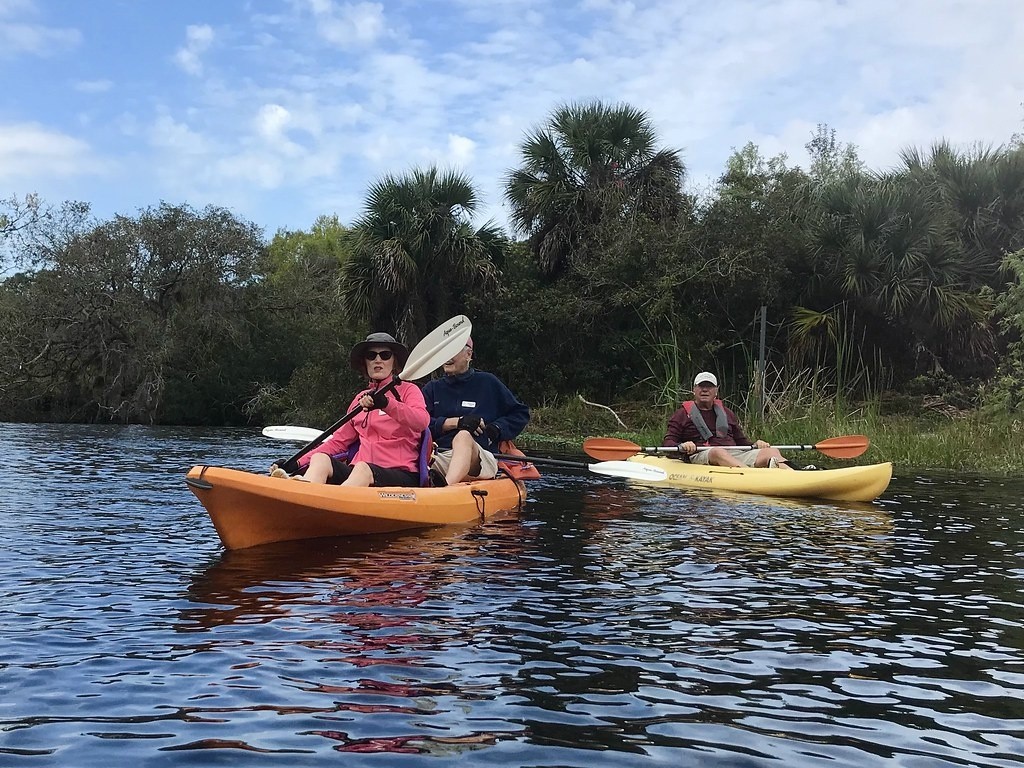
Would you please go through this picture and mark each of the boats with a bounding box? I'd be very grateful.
[186,455,527,552]
[625,448,892,503]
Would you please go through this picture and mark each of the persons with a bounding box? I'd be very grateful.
[269,332,431,487]
[661,372,817,470]
[420,337,529,487]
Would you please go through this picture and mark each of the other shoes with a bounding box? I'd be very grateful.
[768,456,779,468]
[428,468,448,487]
[293,474,304,481]
[802,464,817,470]
[271,468,288,479]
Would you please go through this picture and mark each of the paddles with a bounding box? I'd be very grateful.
[583,434,870,461]
[262,425,666,482]
[267,312,472,476]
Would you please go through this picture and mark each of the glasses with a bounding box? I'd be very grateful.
[364,351,394,360]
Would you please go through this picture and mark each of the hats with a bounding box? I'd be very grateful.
[350,333,408,376]
[466,337,472,348]
[694,372,717,387]
